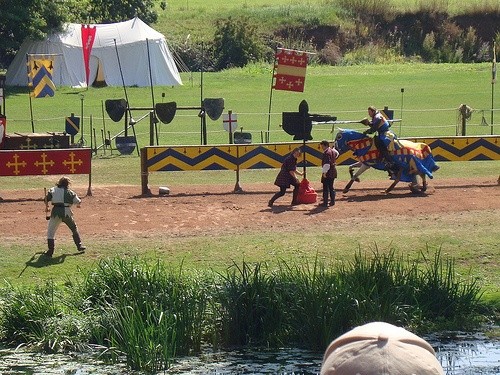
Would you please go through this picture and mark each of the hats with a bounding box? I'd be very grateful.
[320,322,444,375]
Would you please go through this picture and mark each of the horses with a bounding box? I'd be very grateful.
[332,126,441,197]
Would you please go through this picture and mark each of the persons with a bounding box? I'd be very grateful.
[44,176,87,256]
[269,148,304,206]
[362,107,395,166]
[320,141,338,205]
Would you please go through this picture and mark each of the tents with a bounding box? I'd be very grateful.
[4,18,200,87]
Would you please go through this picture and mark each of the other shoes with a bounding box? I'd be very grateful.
[385,162,393,171]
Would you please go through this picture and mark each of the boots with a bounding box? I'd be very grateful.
[269,193,281,207]
[292,190,300,205]
[46,239,54,257]
[73,233,86,251]
